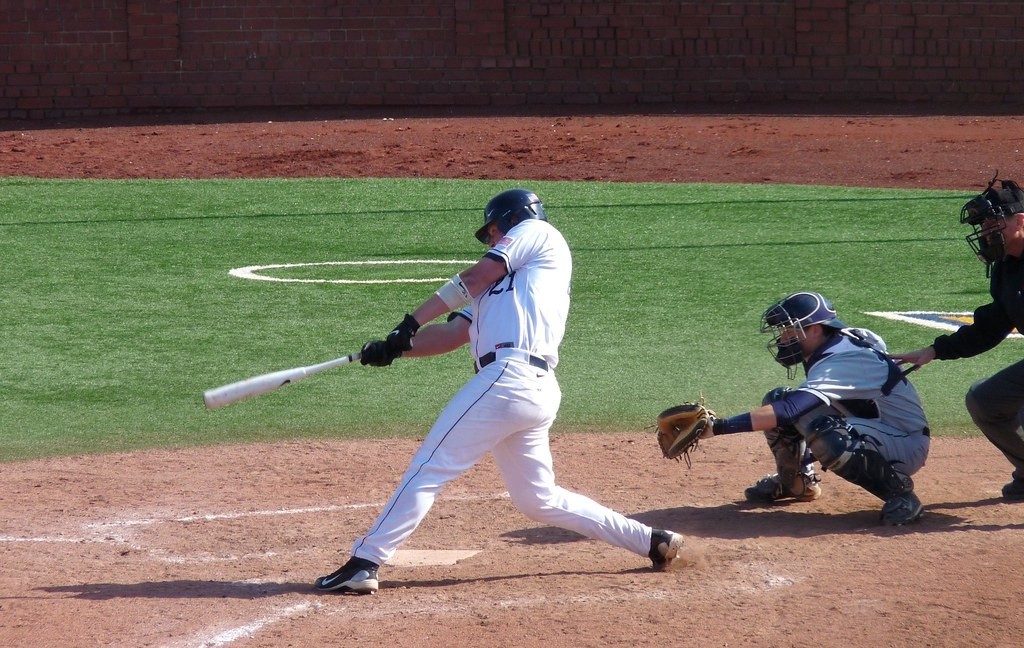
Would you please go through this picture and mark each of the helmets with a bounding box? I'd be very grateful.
[760,291,847,329]
[474,189,548,244]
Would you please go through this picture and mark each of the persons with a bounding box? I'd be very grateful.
[698,291,931,525]
[314,189,684,596]
[888,180,1024,500]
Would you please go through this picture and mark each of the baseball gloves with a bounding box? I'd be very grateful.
[642,390,718,470]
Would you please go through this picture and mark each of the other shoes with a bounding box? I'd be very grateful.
[1002,471,1024,499]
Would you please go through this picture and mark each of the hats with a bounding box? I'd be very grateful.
[960,188,1024,226]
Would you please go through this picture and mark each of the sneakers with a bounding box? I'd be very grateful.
[648,529,683,572]
[315,565,379,595]
[744,476,821,507]
[879,491,924,525]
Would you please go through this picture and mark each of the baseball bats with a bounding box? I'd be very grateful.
[202,337,413,410]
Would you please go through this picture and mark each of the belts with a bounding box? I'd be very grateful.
[474,352,548,375]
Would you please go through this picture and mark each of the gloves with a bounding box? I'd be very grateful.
[360,340,402,366]
[387,313,420,351]
